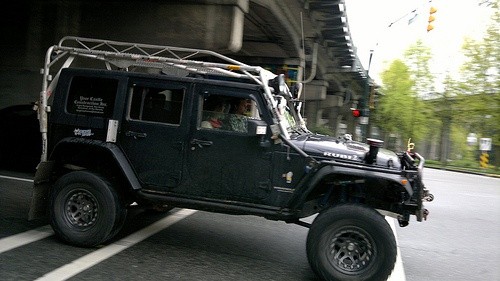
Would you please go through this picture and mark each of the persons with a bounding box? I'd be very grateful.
[222,98,262,133]
[200,98,226,130]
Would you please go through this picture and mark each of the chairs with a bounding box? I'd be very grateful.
[131,84,171,118]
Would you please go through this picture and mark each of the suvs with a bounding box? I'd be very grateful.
[28,35,435,281]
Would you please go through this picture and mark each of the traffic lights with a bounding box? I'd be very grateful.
[353,109,360,117]
